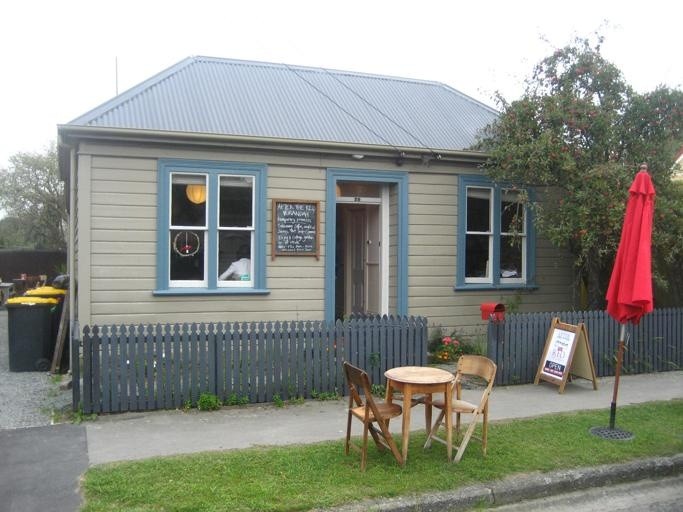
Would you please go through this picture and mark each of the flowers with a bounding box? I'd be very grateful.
[432,337,466,361]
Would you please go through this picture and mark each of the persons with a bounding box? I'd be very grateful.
[217,243,249,281]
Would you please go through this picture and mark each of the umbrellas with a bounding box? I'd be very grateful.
[602,160,655,430]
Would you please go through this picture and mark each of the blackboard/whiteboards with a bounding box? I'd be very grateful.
[270,197,321,261]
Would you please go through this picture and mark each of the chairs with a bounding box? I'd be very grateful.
[341,354,497,472]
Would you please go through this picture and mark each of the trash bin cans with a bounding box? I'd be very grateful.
[4,286,67,372]
